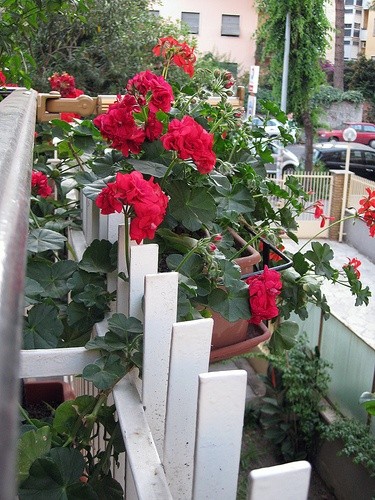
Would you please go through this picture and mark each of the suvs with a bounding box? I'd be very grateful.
[310,142,375,180]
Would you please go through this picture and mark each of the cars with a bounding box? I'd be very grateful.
[246,117,297,145]
[316,122,375,148]
[246,138,299,177]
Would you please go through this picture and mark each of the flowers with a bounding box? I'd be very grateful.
[1,32,375,332]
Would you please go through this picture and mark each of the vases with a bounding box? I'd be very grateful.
[21,381,89,482]
[199,227,260,346]
